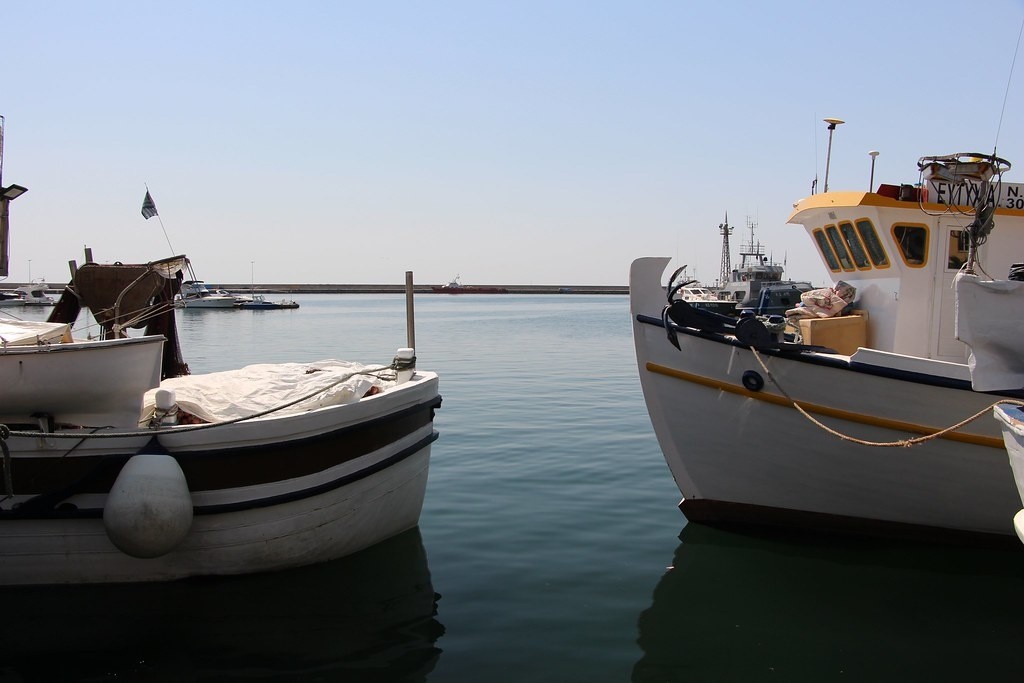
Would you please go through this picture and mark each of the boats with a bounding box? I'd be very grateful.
[431,273,509,293]
[0,116,444,583]
[630,22,1024,541]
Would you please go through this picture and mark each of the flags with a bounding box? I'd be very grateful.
[141,191,158,220]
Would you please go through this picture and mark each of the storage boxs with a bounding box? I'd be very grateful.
[782,310,867,356]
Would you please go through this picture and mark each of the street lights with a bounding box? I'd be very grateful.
[27,259,31,282]
[251,262,254,296]
